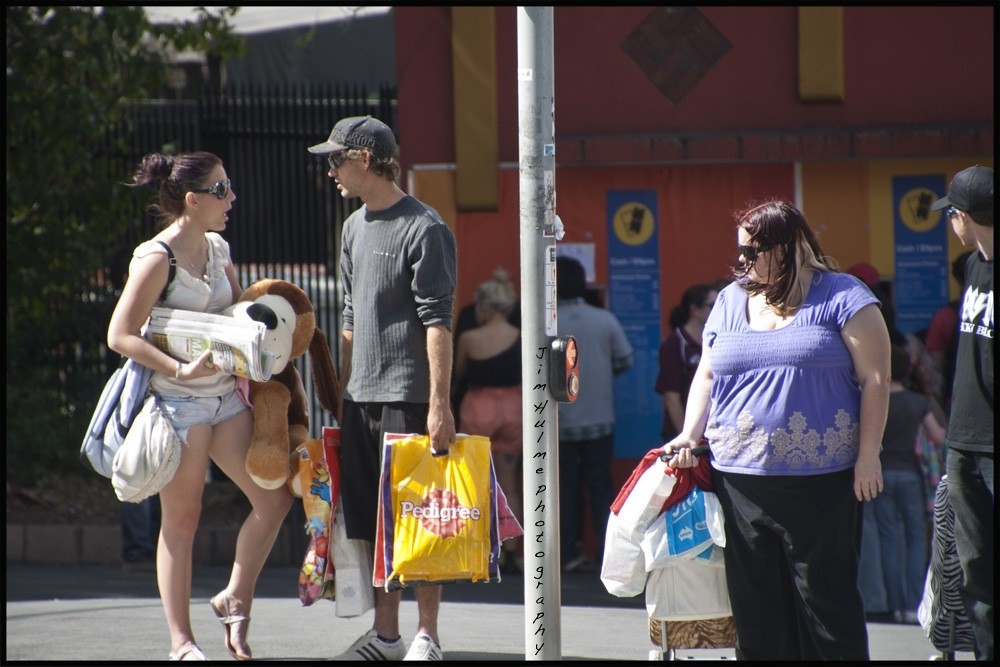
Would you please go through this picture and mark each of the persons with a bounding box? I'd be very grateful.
[106,152,293,661]
[931,164,1000,661]
[664,201,892,661]
[450,257,729,573]
[837,248,977,623]
[306,115,457,661]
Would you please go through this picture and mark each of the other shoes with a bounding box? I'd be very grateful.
[564,549,587,573]
[888,610,904,624]
[904,610,920,624]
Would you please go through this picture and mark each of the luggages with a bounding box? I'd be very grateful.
[916,473,975,661]
[647,444,744,663]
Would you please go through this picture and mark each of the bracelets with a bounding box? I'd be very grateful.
[175,362,180,381]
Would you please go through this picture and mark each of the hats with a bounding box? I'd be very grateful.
[307,115,396,160]
[931,165,994,212]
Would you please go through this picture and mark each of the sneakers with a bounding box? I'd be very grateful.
[401,634,443,661]
[332,628,408,660]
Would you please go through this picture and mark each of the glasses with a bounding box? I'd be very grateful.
[945,207,969,220]
[183,179,231,199]
[702,303,714,308]
[737,239,793,261]
[327,154,348,170]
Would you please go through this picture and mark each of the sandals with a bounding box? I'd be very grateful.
[209,589,252,661]
[170,642,207,662]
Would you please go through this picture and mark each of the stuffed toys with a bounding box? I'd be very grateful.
[219,279,340,498]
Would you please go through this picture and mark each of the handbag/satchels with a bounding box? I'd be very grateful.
[294,428,375,618]
[640,486,727,572]
[372,433,524,594]
[600,455,677,598]
[112,391,182,503]
[80,328,153,479]
[645,559,733,621]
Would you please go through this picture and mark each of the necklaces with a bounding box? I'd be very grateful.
[172,228,211,283]
